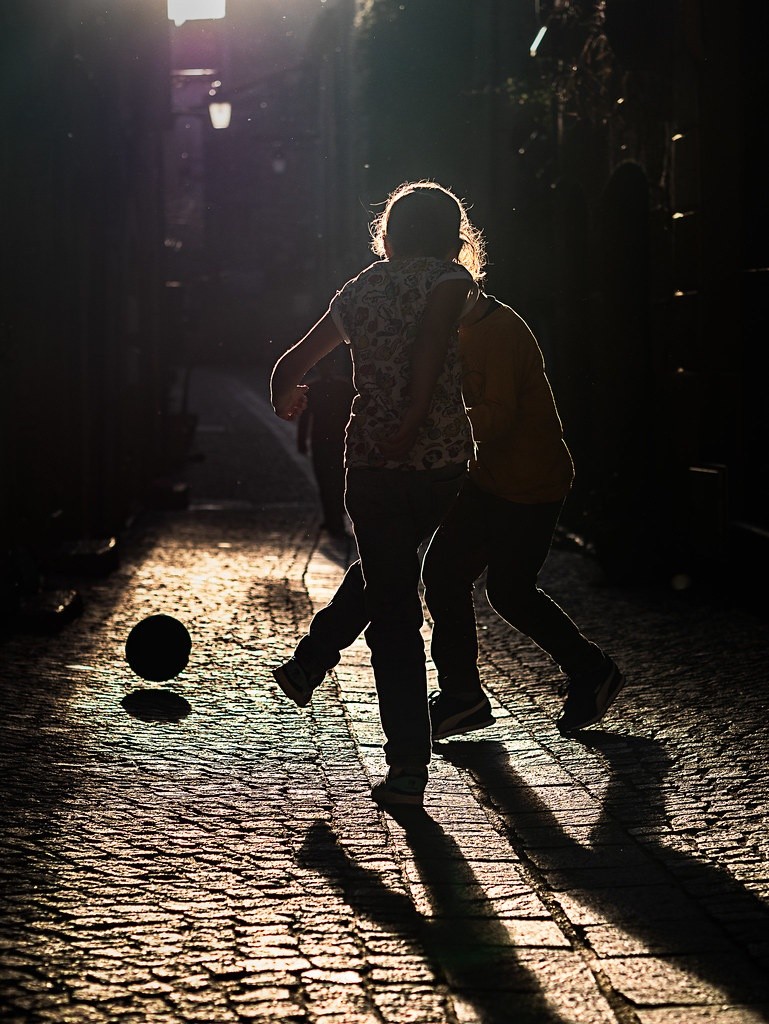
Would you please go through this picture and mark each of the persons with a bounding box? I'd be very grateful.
[270,179,484,812]
[422,284,623,740]
[296,351,357,541]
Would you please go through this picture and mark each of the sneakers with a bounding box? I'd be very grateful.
[273,659,313,706]
[371,767,428,807]
[555,656,626,731]
[428,692,497,741]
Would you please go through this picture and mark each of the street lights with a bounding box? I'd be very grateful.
[149,69,233,495]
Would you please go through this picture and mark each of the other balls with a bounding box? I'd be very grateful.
[125,614,192,681]
